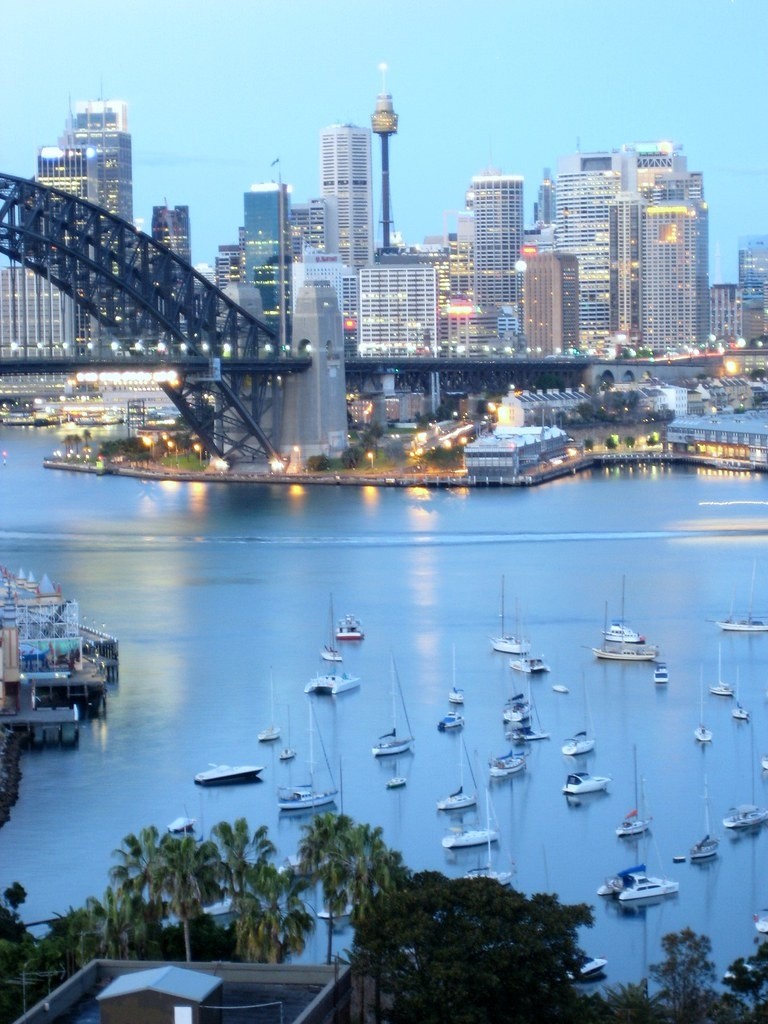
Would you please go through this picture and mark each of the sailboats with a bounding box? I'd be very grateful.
[592,570,674,686]
[260,672,281,742]
[707,637,735,699]
[508,609,552,673]
[714,556,768,631]
[449,647,467,706]
[435,733,482,813]
[596,773,682,908]
[302,588,361,701]
[276,698,340,811]
[728,665,750,719]
[462,788,515,887]
[486,573,533,656]
[693,664,726,750]
[721,719,768,830]
[688,770,725,862]
[370,652,430,760]
[614,743,654,837]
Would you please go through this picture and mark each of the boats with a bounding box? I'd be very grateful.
[274,851,330,882]
[562,730,600,761]
[436,710,466,732]
[191,763,264,789]
[204,896,241,917]
[317,904,355,919]
[754,910,768,935]
[562,771,613,797]
[558,950,610,987]
[335,612,367,643]
[387,777,411,790]
[169,814,197,836]
[722,961,757,983]
